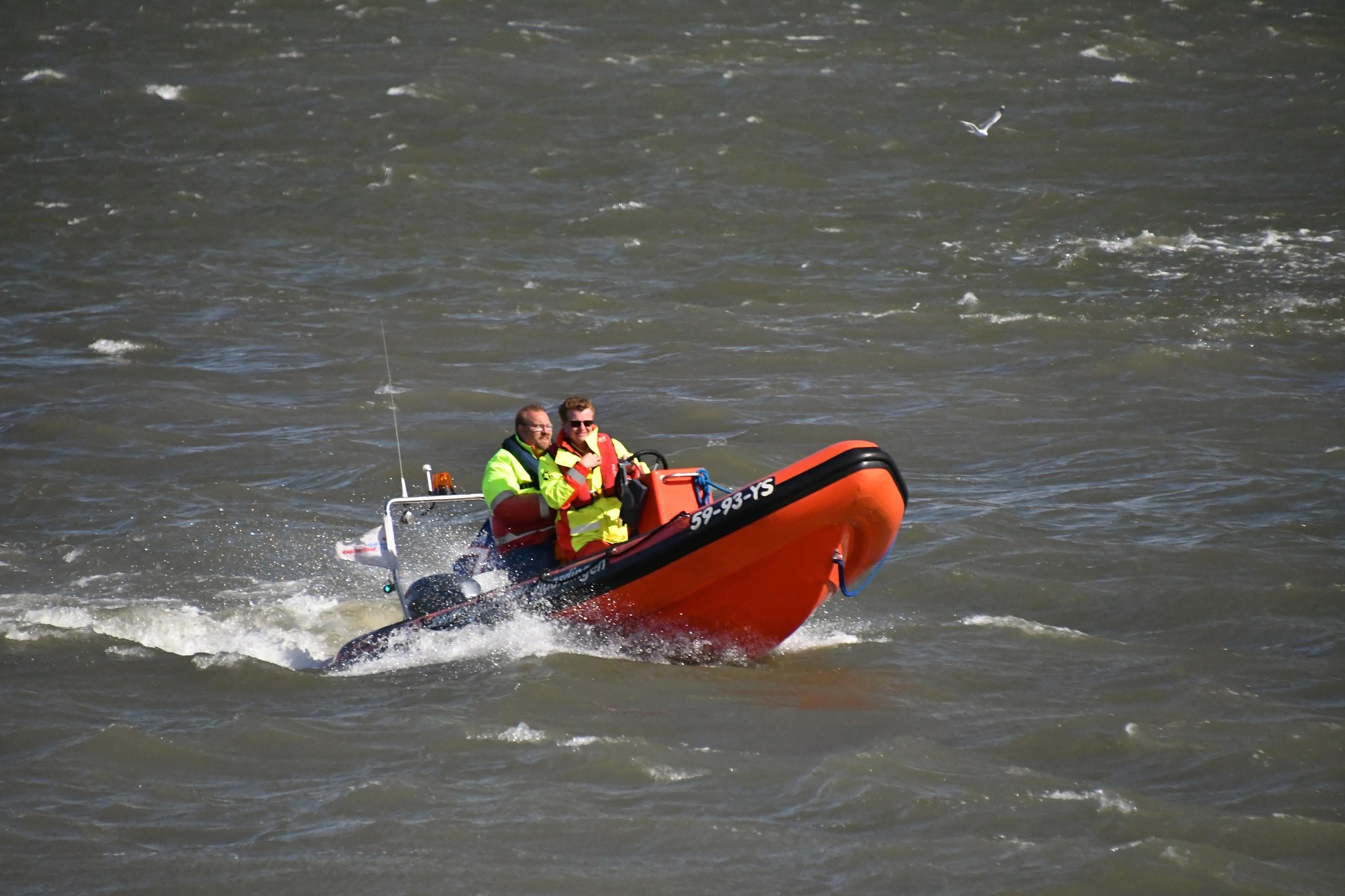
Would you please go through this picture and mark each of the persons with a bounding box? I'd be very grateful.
[481,404,554,586]
[538,396,650,565]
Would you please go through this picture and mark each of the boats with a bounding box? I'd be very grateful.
[312,439,911,675]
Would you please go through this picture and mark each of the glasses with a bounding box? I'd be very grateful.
[567,420,593,428]
[524,422,555,432]
